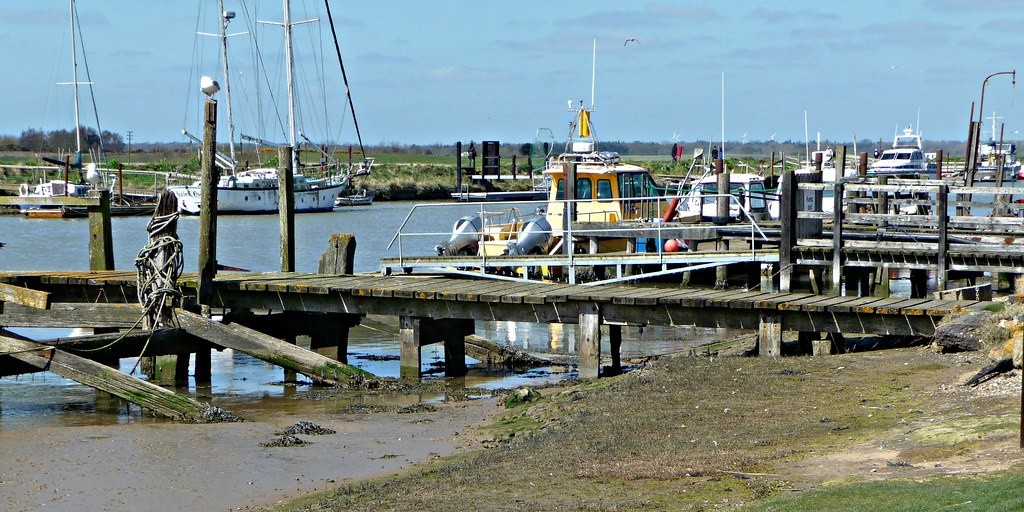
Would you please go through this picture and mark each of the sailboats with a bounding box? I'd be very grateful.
[20,0,168,218]
[162,0,375,216]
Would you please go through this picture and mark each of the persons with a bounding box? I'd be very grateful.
[711,146,723,162]
[671,142,683,163]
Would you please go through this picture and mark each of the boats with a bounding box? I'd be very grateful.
[432,36,938,282]
[333,186,375,206]
[972,111,1023,184]
